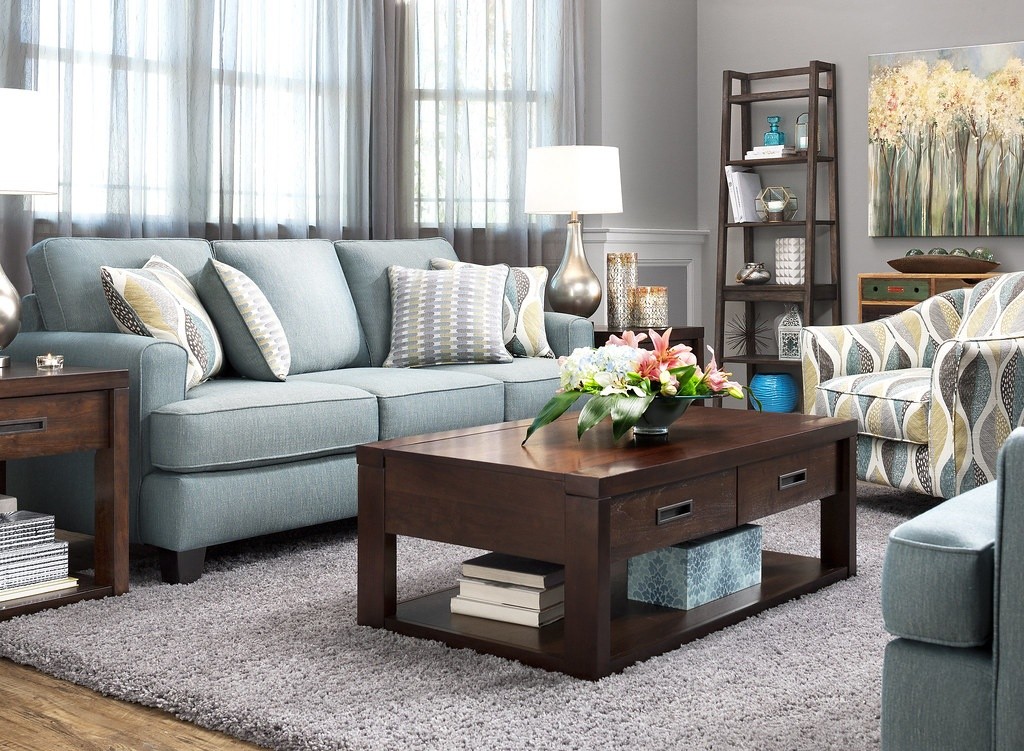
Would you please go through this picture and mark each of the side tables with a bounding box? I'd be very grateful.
[594,325,706,406]
[0,360,130,621]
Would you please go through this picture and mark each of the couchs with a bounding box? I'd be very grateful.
[880,425,1023,750]
[798,269,1024,502]
[0,236,595,586]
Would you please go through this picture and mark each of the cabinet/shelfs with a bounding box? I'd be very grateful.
[715,58,841,418]
[856,272,1009,325]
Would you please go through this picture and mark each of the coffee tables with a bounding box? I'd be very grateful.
[355,408,858,683]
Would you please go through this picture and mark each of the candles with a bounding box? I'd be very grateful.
[767,201,783,209]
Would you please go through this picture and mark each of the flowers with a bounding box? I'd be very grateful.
[521,328,762,446]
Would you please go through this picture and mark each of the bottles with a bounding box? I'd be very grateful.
[763,116,785,146]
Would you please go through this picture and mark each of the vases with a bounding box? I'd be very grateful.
[628,393,730,435]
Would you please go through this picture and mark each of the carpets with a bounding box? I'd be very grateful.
[0,482,948,751]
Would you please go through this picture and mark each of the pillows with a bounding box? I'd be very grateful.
[510,265,556,359]
[381,263,513,367]
[100,253,224,394]
[196,257,291,383]
[431,257,535,358]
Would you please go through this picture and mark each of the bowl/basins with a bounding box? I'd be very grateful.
[748,372,799,413]
[886,254,1000,273]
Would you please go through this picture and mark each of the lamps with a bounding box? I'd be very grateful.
[0,86,61,368]
[795,112,821,156]
[525,145,624,328]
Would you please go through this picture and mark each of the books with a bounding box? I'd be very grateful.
[0,509,79,602]
[448,553,565,628]
[725,165,764,223]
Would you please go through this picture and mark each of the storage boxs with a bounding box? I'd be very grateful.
[628,523,761,610]
[0,494,68,589]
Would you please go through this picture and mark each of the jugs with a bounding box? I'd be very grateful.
[735,262,771,286]
